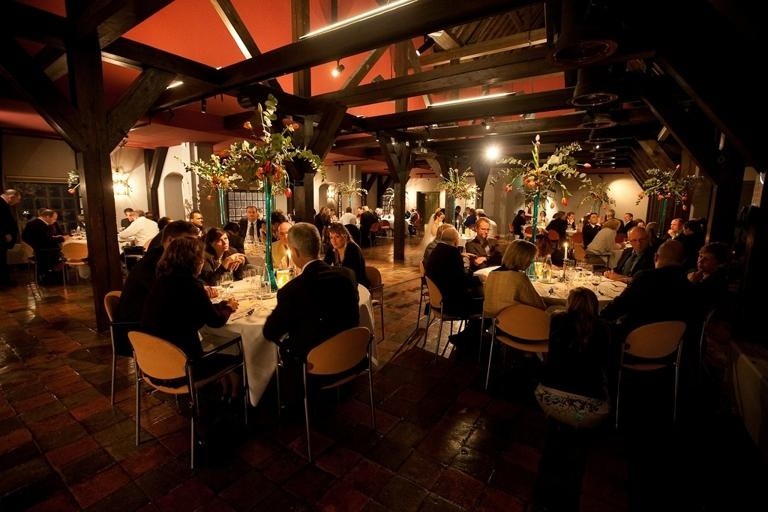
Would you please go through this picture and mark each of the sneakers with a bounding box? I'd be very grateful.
[448,334,466,350]
[220,394,243,407]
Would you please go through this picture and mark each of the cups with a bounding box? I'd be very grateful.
[535,262,543,278]
[216,268,294,307]
[563,259,602,292]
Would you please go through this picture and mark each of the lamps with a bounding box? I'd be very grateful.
[569,58,620,108]
[543,0,619,70]
[109,164,135,198]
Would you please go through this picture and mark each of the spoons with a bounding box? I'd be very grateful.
[549,287,553,296]
[231,309,255,322]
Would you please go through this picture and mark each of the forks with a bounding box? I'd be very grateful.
[609,283,624,292]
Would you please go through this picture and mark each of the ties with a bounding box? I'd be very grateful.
[623,253,638,273]
[249,222,255,240]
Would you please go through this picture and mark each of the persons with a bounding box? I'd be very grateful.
[22,208,72,270]
[314,205,381,257]
[238,206,300,271]
[536,285,612,430]
[0,187,22,289]
[542,209,727,365]
[117,206,266,286]
[422,206,548,368]
[112,220,242,407]
[406,209,420,233]
[263,222,366,405]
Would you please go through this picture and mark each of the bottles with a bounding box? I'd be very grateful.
[543,254,552,281]
